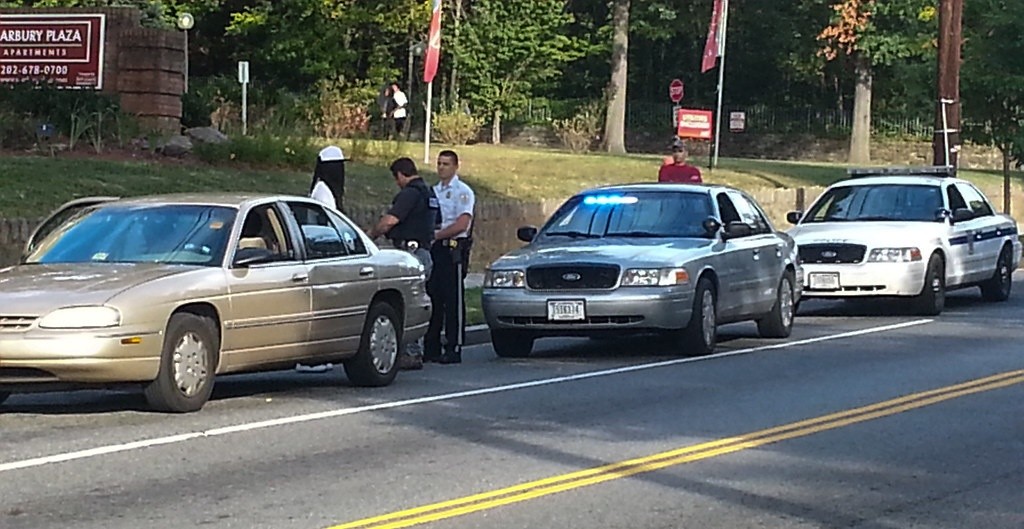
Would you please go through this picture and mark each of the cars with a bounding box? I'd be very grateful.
[783,164,1023,318]
[0,190,433,415]
[479,183,805,355]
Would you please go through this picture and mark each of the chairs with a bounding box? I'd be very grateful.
[239,210,271,250]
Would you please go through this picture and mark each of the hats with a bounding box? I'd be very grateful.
[317,146,353,163]
[671,140,686,148]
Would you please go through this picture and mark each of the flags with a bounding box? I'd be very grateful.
[424,0,441,83]
[701,0,725,72]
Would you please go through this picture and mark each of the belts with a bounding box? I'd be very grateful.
[442,239,459,248]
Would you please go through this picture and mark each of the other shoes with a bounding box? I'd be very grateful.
[414,349,442,361]
[297,363,333,373]
[438,351,462,364]
[398,352,423,370]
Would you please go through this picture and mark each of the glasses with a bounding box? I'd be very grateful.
[673,149,683,153]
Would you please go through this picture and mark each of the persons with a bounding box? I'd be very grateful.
[368,157,442,370]
[658,140,702,183]
[380,87,398,140]
[422,150,475,365]
[391,83,408,133]
[296,146,349,371]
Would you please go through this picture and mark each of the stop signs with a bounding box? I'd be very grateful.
[669,79,684,102]
